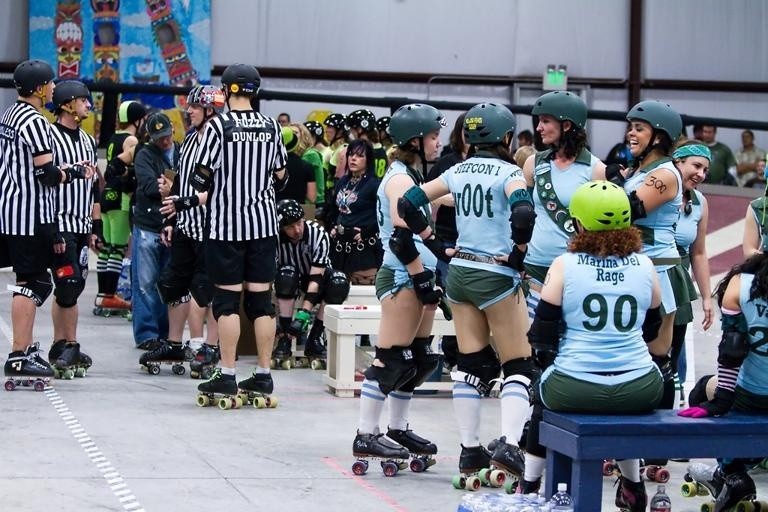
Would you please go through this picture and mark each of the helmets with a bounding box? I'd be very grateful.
[391,102,448,146]
[463,102,516,144]
[532,91,587,130]
[569,178,631,232]
[627,99,683,142]
[275,198,305,227]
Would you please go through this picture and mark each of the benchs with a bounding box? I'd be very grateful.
[537,409,768,511]
[322,300,459,397]
[270,283,379,359]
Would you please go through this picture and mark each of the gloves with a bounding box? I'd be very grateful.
[677,387,736,418]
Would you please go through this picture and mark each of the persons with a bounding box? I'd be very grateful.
[45,81,103,380]
[676,249,767,511]
[516,180,665,511]
[397,102,542,494]
[601,99,684,482]
[352,103,448,477]
[1,60,98,391]
[94,61,767,411]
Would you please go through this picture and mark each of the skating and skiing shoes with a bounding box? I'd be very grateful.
[352,425,409,476]
[480,436,524,492]
[386,424,438,471]
[452,443,492,491]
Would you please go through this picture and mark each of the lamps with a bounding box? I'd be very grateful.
[541,61,571,94]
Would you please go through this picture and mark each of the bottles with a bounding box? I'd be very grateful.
[650,485,671,512]
[458,480,576,512]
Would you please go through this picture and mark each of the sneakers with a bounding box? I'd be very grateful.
[604,459,767,511]
